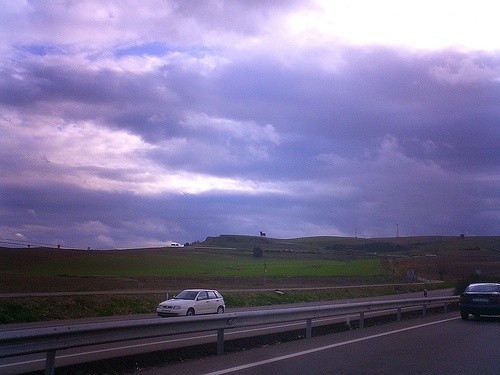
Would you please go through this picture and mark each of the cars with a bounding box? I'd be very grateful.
[169,242,184,248]
[157,288,226,316]
[458,283,500,320]
